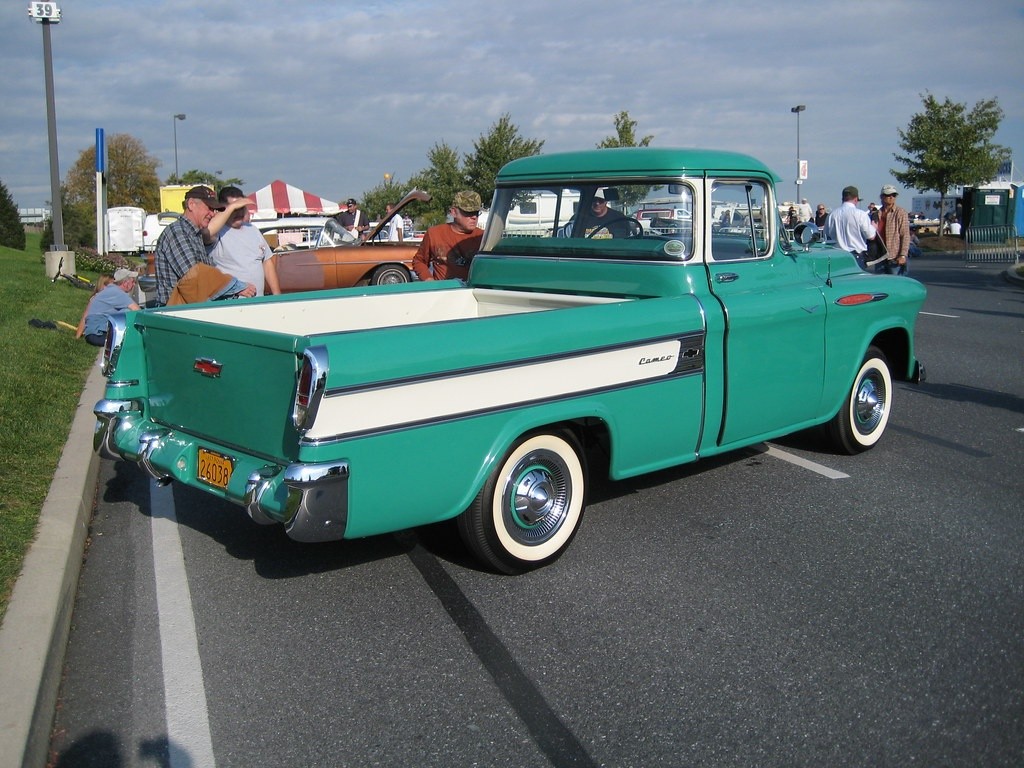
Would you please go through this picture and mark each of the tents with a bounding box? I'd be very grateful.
[243,180,348,221]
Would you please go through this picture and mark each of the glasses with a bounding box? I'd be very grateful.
[819,208,824,210]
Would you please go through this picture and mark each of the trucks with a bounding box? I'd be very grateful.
[777,203,812,226]
[649,216,692,234]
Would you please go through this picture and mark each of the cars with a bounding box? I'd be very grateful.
[363,222,423,241]
[138,185,434,296]
[674,208,692,220]
[632,209,673,235]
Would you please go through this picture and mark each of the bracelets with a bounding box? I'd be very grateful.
[902,256,905,258]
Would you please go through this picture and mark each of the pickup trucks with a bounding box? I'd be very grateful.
[93,146,928,575]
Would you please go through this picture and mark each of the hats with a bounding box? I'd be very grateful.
[594,189,605,199]
[842,186,864,201]
[346,199,356,205]
[114,269,138,282]
[185,185,226,212]
[881,185,899,195]
[453,190,482,212]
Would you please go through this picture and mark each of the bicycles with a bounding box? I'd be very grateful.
[54,257,96,290]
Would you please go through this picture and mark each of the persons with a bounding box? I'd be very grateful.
[569,189,630,240]
[815,204,828,227]
[76,269,141,347]
[786,206,798,229]
[386,203,403,242]
[412,191,484,282]
[202,187,282,301]
[908,227,920,258]
[154,185,257,308]
[798,198,812,222]
[823,186,878,271]
[868,203,877,215]
[870,184,910,277]
[337,199,369,240]
[950,219,961,238]
[917,212,925,219]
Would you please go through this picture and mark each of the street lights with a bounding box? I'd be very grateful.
[173,114,186,184]
[214,170,222,191]
[791,105,806,204]
